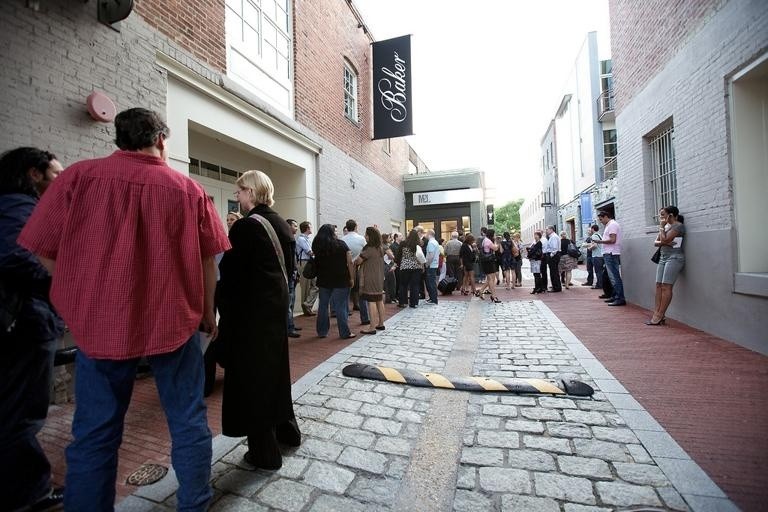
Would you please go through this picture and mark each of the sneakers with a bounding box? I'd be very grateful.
[391,296,438,308]
[349,332,357,338]
[288,326,302,338]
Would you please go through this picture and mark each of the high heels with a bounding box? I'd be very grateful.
[644,318,666,325]
[461,288,502,303]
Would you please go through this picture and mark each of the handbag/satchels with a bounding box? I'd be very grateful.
[512,245,519,257]
[303,256,317,279]
[526,247,542,259]
[650,249,661,264]
[567,243,581,258]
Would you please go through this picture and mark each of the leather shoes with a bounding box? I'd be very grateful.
[360,321,385,335]
[582,281,593,286]
[591,285,602,289]
[599,294,626,305]
[531,282,573,294]
[27,487,66,510]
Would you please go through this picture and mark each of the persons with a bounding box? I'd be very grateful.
[644,206,685,325]
[17,107,234,512]
[227,211,243,230]
[1,145,68,511]
[594,211,626,305]
[286,219,615,336]
[218,170,301,471]
[204,253,224,399]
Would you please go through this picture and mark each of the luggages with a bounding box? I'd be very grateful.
[437,266,461,296]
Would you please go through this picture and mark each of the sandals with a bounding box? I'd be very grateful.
[497,279,522,290]
